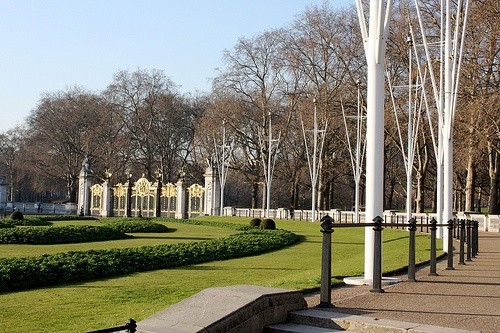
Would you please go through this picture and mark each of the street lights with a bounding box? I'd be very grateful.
[338,78,366,224]
[299,96,329,223]
[256,109,282,219]
[386,30,426,231]
[212,116,235,219]
